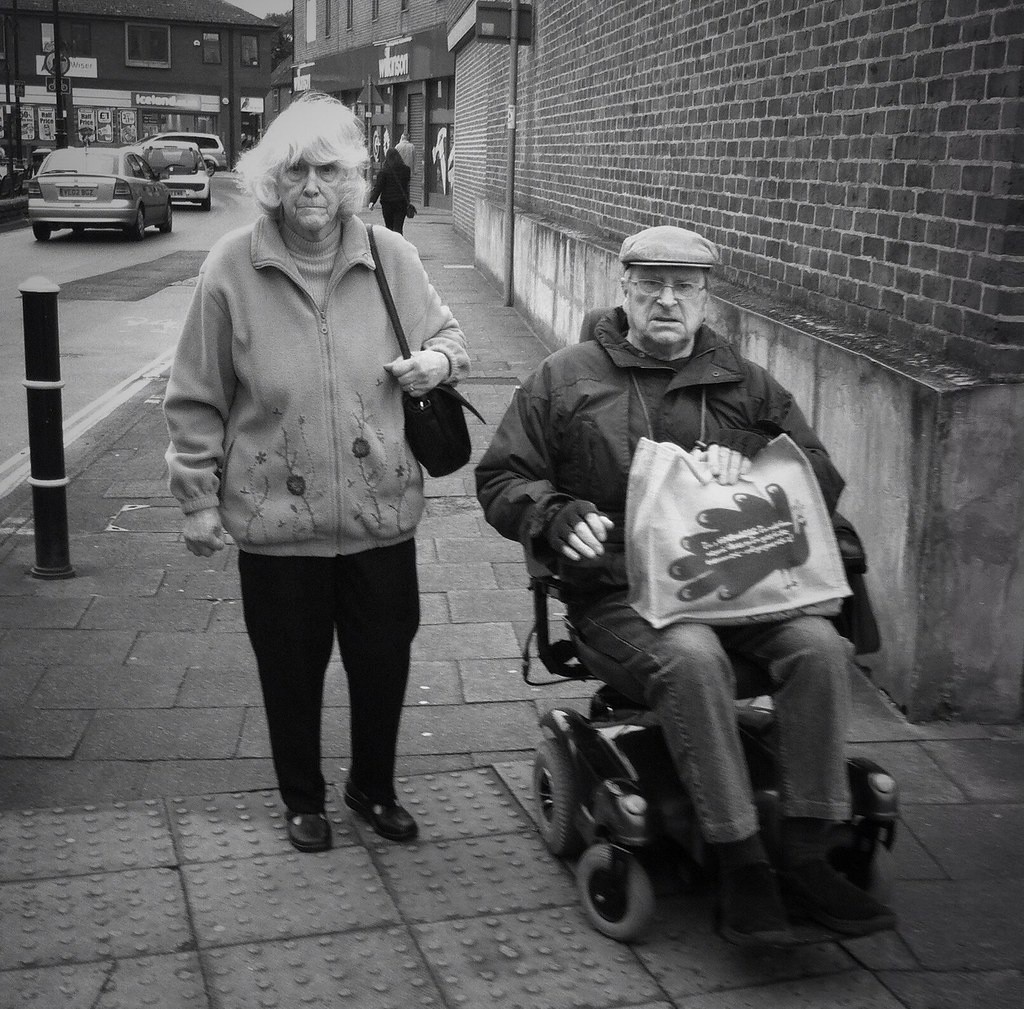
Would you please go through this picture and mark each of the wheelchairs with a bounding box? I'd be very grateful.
[519,538,897,952]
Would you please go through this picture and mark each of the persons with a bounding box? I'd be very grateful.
[165,93,472,852]
[368,148,411,236]
[395,134,416,174]
[475,226,900,963]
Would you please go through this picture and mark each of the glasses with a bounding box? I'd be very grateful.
[626,279,707,301]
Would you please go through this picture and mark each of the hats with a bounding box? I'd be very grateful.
[618,225,723,269]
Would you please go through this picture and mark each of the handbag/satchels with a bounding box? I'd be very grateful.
[407,203,418,218]
[626,434,853,630]
[403,383,486,478]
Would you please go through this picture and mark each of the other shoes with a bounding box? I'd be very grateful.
[721,861,794,947]
[781,844,901,935]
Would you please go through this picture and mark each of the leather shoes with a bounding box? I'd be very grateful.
[343,777,419,841]
[284,808,333,852]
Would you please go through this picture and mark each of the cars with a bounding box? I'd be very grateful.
[138,139,214,211]
[0,147,25,200]
[111,132,228,176]
[26,146,172,243]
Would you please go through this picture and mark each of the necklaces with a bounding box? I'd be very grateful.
[627,368,707,451]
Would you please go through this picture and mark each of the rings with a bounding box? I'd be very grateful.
[410,384,416,391]
